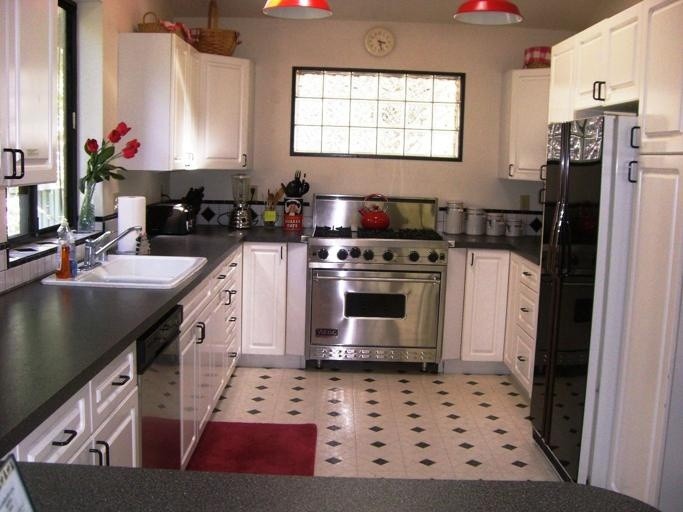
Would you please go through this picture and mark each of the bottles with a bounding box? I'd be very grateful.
[445,200,521,237]
[263,210,277,230]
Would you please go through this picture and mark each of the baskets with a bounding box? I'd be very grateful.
[138,11,166,32]
[194,1,242,55]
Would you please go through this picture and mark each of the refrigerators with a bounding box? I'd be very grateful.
[530,111,639,489]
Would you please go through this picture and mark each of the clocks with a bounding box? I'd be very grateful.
[364,27,394,57]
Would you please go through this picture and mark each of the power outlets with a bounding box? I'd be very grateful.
[519,195,529,210]
[248,184,259,200]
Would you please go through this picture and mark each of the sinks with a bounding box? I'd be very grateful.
[72,254,208,290]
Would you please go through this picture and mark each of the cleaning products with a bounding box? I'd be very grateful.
[55,219,69,279]
[68,229,77,278]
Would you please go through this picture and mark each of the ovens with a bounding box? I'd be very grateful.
[307,270,448,371]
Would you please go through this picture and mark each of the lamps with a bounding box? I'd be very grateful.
[453,1,524,25]
[260,0,333,20]
[610,155,683,508]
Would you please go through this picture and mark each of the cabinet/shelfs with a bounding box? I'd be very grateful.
[243,238,288,356]
[496,67,549,183]
[201,53,255,170]
[17,341,141,469]
[117,33,201,172]
[177,272,223,472]
[506,255,541,396]
[638,0,683,153]
[575,2,643,110]
[462,242,511,361]
[1,2,57,189]
[221,245,243,387]
[548,38,575,122]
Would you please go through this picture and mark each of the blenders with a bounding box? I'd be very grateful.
[229,173,253,229]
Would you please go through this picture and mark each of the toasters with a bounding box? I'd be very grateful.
[146,200,195,235]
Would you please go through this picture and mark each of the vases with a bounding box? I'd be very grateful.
[79,181,95,233]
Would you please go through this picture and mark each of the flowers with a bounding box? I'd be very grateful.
[78,123,140,224]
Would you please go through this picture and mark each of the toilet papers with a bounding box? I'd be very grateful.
[117,196,147,254]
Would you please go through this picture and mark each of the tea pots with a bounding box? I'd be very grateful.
[358,194,390,231]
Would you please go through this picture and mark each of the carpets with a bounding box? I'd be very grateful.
[183,421,317,477]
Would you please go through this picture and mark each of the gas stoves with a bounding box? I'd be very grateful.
[307,225,448,271]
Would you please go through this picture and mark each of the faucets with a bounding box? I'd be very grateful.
[94,225,143,258]
